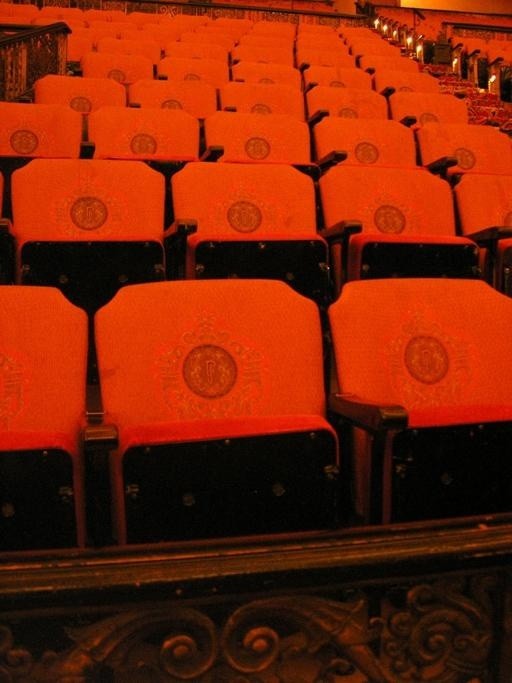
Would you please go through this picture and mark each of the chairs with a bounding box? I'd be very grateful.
[1,0,512,556]
